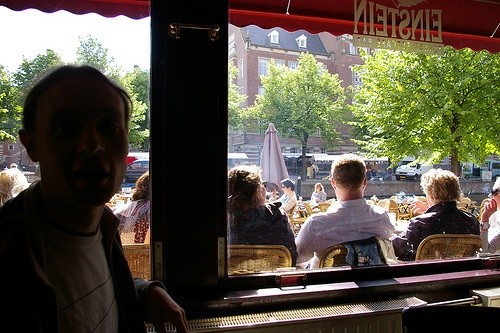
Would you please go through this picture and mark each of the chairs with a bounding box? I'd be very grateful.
[121,196,491,281]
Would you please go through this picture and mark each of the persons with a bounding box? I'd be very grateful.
[227,165,298,267]
[398,169,480,261]
[478,177,500,261]
[274,179,327,214]
[295,153,396,269]
[0,66,189,333]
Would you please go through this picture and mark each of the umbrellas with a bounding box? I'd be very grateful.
[258,122,290,187]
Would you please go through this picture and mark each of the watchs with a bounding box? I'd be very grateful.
[479,221,490,231]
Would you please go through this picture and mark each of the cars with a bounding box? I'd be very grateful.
[371,173,392,182]
[121,159,149,185]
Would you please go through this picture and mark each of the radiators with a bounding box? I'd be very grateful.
[146,288,500,333]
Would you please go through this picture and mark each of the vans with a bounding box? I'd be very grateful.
[395,161,433,181]
[480,160,500,181]
